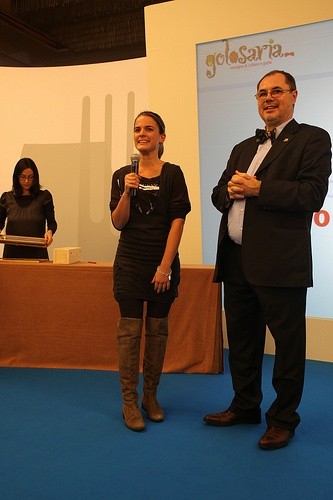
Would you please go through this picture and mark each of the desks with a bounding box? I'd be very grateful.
[0,261,224,375]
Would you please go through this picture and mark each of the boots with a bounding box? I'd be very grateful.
[141,318,168,422]
[116,318,145,430]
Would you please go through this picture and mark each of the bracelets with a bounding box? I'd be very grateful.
[157,266,172,280]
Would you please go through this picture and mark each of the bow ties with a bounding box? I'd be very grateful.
[255,128,276,145]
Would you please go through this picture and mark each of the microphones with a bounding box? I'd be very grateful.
[130,154,141,196]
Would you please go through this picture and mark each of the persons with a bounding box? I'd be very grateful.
[0,158,58,261]
[109,111,191,432]
[204,70,332,450]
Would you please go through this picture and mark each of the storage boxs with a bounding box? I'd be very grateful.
[53,246,81,264]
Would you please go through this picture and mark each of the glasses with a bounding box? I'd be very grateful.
[254,89,291,100]
[18,176,33,181]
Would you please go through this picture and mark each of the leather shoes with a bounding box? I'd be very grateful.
[257,426,294,450]
[203,408,252,427]
